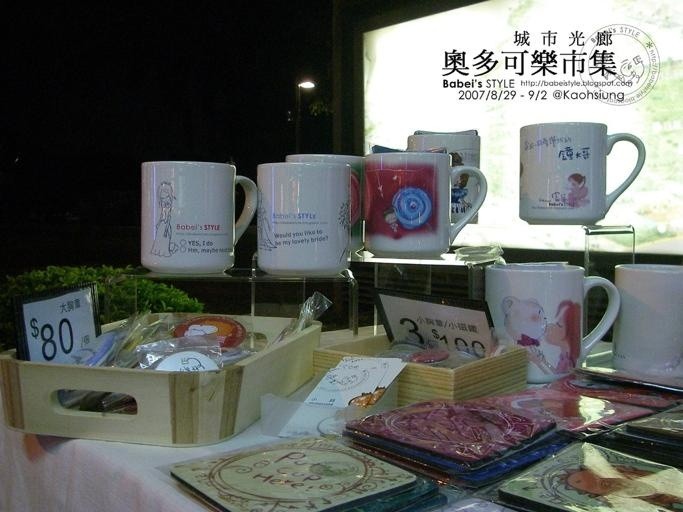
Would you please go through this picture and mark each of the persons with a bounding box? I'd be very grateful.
[567,173,589,208]
[528,300,581,375]
[150,181,173,257]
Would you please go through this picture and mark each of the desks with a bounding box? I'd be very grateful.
[5,318,678,510]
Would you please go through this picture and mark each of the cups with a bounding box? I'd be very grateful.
[140,122,646,274]
[483,265,682,385]
